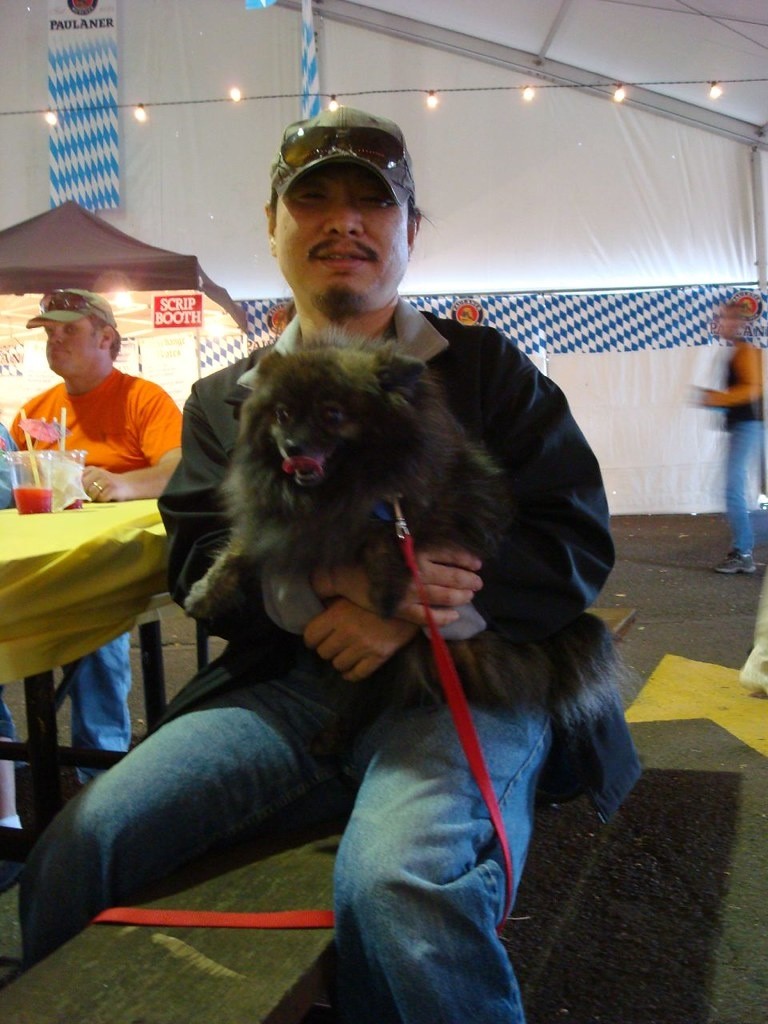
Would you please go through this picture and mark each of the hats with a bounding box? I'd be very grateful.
[25,288,117,331]
[270,105,416,206]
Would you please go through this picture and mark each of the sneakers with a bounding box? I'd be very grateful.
[714,551,756,571]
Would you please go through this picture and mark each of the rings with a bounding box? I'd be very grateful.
[93,481,103,492]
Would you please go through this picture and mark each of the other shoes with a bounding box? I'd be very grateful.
[739,651,768,697]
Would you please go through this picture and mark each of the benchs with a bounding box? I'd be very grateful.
[0,604,641,1024]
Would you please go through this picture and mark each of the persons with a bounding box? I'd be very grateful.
[692,304,768,573]
[0,288,185,860]
[18,106,641,1024]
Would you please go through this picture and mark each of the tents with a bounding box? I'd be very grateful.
[0,200,254,415]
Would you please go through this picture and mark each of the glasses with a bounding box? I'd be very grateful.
[39,292,108,321]
[277,126,414,188]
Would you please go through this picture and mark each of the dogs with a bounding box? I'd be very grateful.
[184,339,628,719]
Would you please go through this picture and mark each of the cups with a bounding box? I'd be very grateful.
[41,450,85,508]
[6,449,52,515]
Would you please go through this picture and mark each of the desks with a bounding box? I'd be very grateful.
[0,498,176,892]
